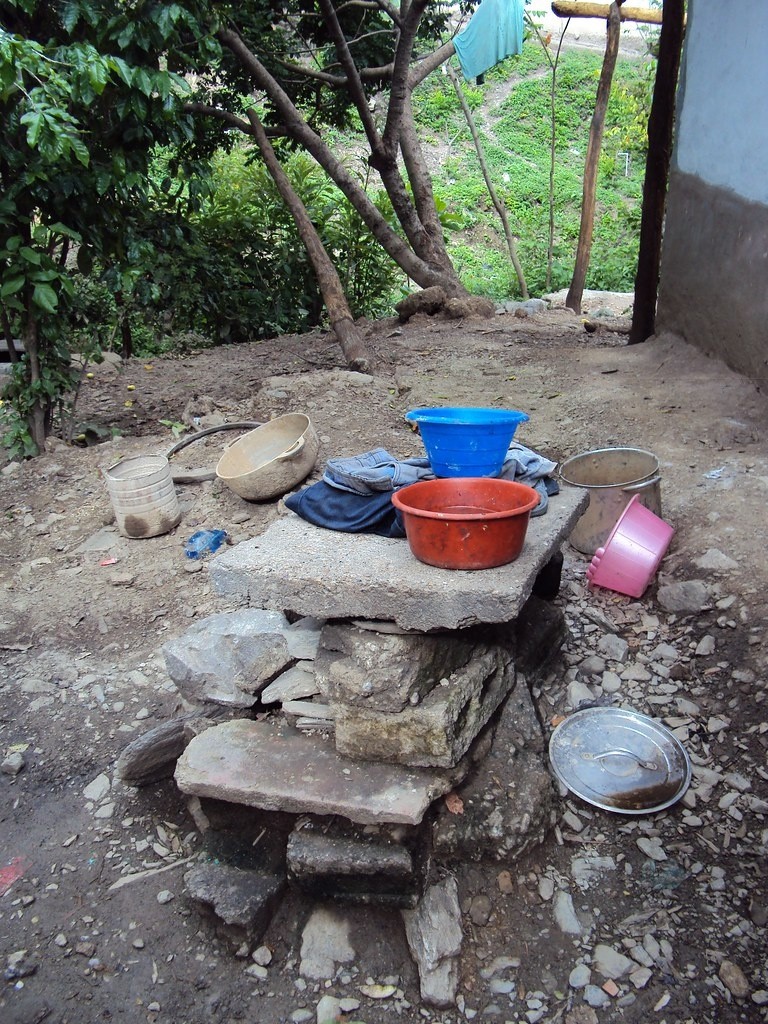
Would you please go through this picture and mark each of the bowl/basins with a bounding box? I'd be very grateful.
[391,477,540,568]
[586,493,675,598]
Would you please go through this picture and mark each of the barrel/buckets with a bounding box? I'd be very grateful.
[408,408,529,478]
[105,455,181,539]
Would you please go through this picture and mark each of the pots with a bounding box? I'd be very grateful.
[559,447,661,556]
[217,412,319,502]
